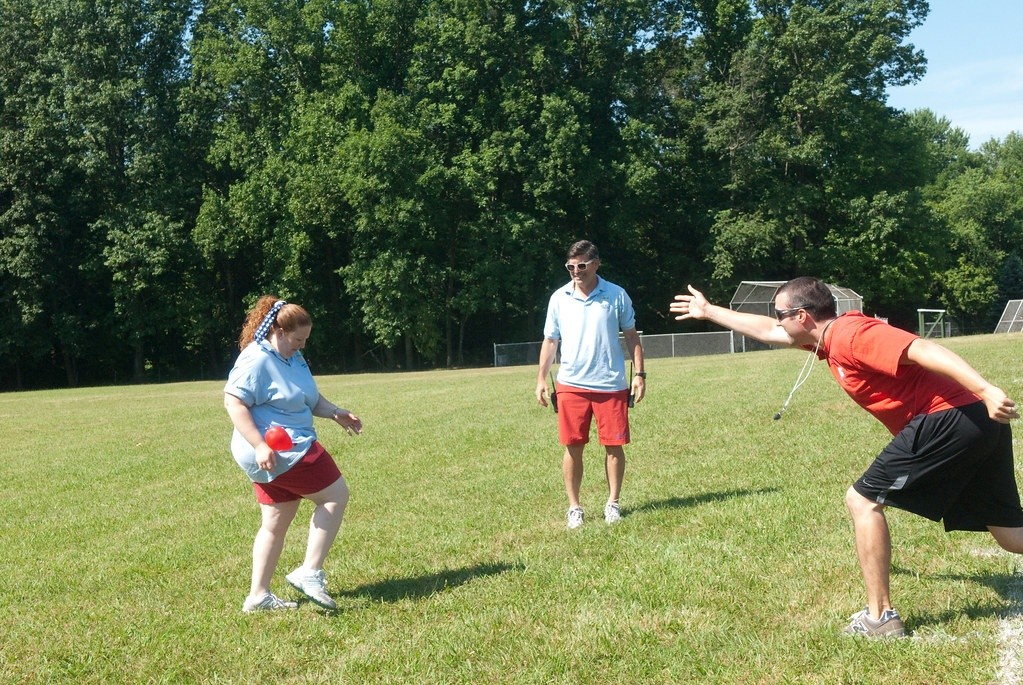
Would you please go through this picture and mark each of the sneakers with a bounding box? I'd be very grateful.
[285,565,337,609]
[565,506,584,528]
[845,605,906,638]
[242,592,297,613]
[604,501,620,524]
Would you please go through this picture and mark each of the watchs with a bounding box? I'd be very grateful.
[635,373,646,379]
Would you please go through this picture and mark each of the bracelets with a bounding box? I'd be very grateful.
[331,407,339,420]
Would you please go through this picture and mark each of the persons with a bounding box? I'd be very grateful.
[534,240,646,530]
[670,277,1023,637]
[223,296,362,612]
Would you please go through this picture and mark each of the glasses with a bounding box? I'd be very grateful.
[775,305,812,321]
[564,259,596,272]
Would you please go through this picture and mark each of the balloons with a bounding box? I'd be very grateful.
[266,427,293,452]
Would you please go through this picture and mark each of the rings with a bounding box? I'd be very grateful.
[1007,407,1012,413]
